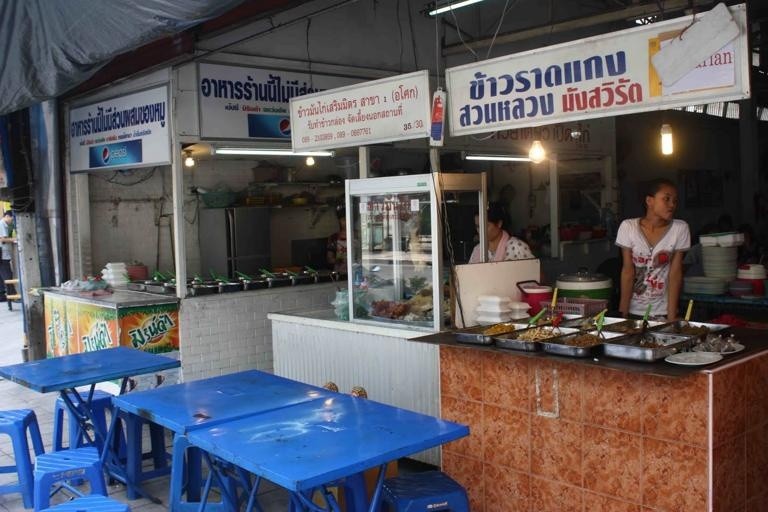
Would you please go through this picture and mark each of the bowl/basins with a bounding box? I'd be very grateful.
[453,321,537,345]
[538,328,627,358]
[518,313,586,326]
[126,269,338,297]
[602,331,691,364]
[702,245,739,285]
[602,318,667,332]
[490,324,582,352]
[556,316,627,330]
[657,318,731,338]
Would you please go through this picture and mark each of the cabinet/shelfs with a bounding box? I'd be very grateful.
[38,286,179,392]
[344,173,488,333]
[268,302,768,512]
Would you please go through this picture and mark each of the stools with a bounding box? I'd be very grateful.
[0,407,45,511]
[33,444,107,512]
[37,493,131,512]
[381,469,468,511]
[51,390,129,467]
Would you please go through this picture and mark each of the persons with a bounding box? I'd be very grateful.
[615,179,691,324]
[0,210,20,300]
[327,207,361,280]
[467,206,535,263]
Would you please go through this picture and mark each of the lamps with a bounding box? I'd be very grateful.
[660,117,674,155]
[570,121,582,139]
[216,147,335,159]
[464,154,532,162]
[428,0,483,17]
[305,156,315,167]
[184,150,195,167]
[528,130,546,164]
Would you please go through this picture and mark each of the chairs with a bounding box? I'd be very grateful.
[113,405,176,500]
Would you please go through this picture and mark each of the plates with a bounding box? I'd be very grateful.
[691,341,746,356]
[664,351,724,366]
[367,313,435,328]
[682,277,725,295]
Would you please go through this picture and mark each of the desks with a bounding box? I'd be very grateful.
[110,369,337,437]
[187,395,469,511]
[0,345,181,499]
